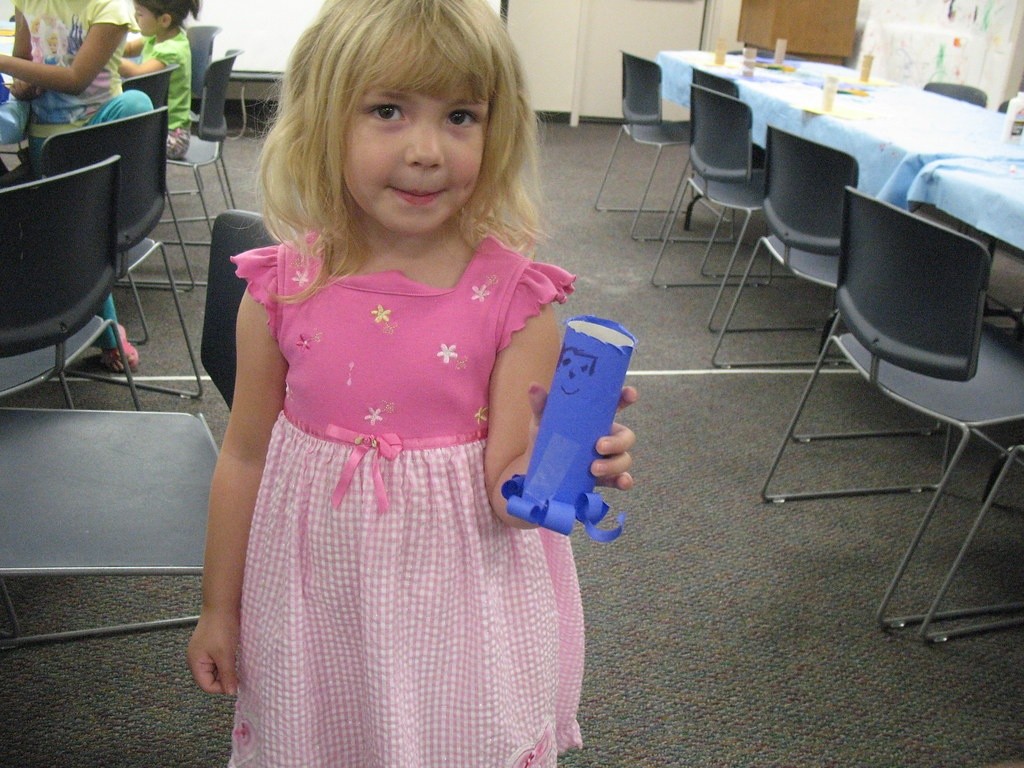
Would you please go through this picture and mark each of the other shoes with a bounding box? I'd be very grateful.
[102,323,138,374]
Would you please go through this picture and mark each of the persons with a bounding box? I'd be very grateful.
[0,0,155,372]
[119,0,200,160]
[187,0,636,767]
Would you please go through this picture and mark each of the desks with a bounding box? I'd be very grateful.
[906,156,1024,261]
[654,51,885,234]
[731,79,1024,211]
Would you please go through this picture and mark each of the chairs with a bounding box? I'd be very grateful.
[594,48,1024,642]
[0,25,287,651]
